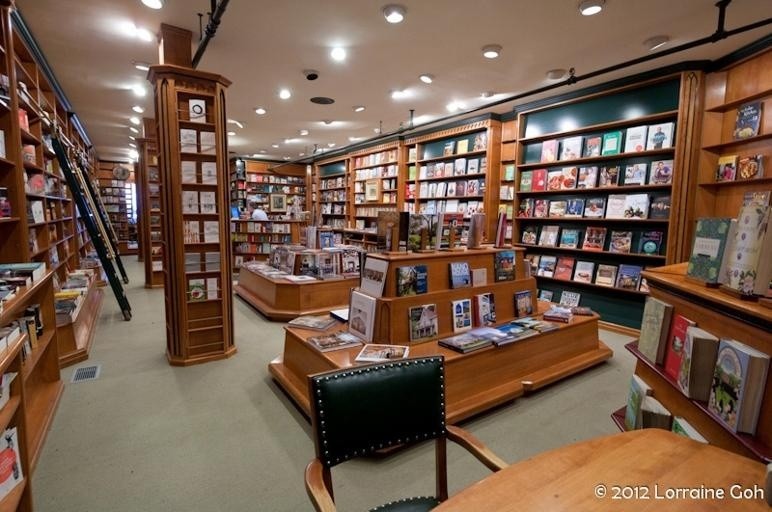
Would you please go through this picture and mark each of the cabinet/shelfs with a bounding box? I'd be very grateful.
[90,138,162,291]
[149,66,239,365]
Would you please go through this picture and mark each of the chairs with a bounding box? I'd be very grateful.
[298,361,509,509]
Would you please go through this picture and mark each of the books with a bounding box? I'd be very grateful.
[287,315,337,332]
[732,100,763,141]
[310,212,592,364]
[516,120,674,293]
[310,133,485,229]
[188,98,206,124]
[104,127,310,303]
[1,74,103,504]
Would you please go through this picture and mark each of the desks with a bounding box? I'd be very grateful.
[429,425,772,510]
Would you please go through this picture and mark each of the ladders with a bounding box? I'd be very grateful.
[51,136,133,321]
